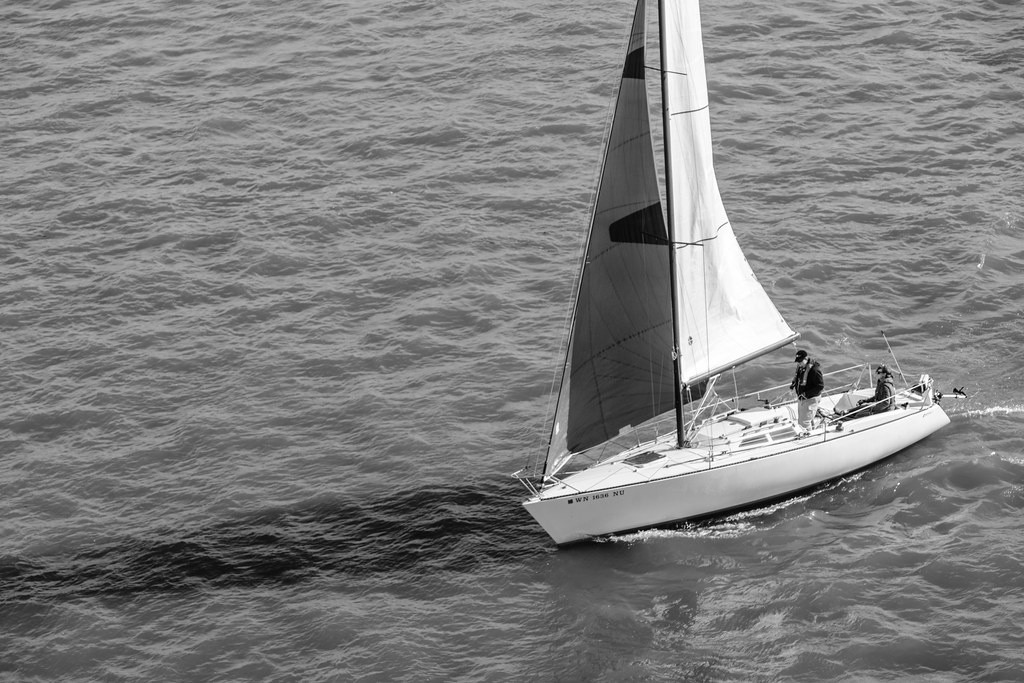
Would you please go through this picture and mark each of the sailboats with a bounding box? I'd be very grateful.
[513,0,972,551]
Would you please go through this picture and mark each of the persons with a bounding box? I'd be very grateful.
[790,350,825,430]
[843,365,896,418]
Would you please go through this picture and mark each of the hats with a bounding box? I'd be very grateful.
[795,351,807,362]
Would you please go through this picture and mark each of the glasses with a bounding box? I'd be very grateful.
[878,372,884,374]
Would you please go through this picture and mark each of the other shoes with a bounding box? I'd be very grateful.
[834,407,847,415]
